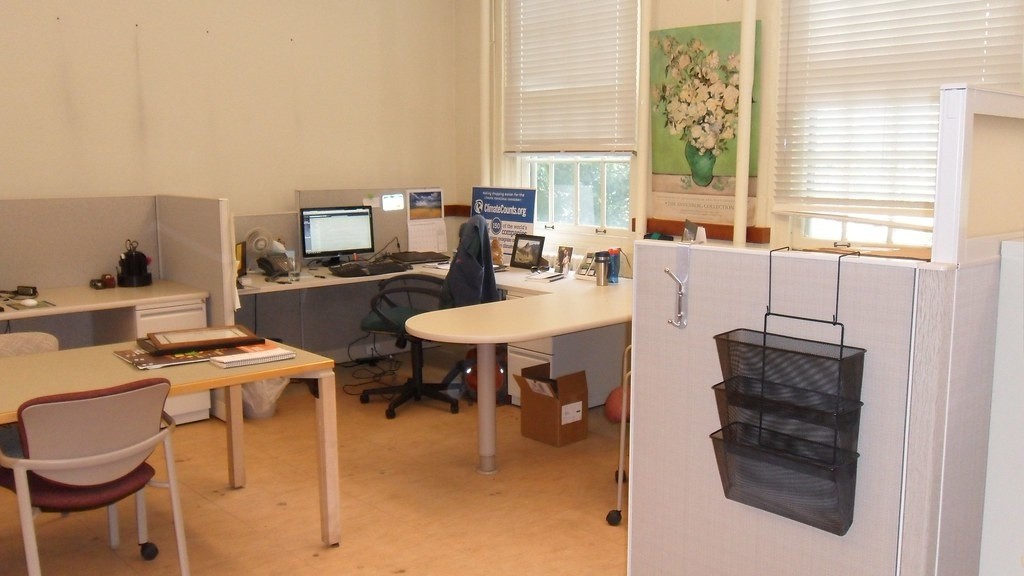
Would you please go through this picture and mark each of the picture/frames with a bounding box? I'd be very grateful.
[148,324,265,350]
[137,337,265,355]
[510,234,545,269]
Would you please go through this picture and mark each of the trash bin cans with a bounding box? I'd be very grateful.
[241,377,280,420]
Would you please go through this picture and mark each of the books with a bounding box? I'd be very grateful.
[527,271,564,282]
[210,347,297,369]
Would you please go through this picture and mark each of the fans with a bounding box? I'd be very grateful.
[245,227,285,256]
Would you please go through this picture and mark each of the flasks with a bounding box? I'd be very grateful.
[595,251,609,286]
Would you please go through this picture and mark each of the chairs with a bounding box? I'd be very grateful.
[0,378,191,576]
[0,332,59,360]
[361,215,499,420]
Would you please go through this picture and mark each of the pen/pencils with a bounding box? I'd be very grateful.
[315,275,326,279]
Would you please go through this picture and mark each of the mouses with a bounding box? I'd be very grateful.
[404,264,413,270]
[21,298,38,307]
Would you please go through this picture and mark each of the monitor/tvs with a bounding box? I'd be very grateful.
[299,205,374,266]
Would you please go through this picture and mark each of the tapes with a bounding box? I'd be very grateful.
[105,275,110,278]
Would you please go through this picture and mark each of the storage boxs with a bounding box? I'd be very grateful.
[513,363,589,447]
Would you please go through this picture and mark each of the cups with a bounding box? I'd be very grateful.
[288,257,301,282]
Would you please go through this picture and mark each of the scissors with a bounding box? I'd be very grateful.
[125,239,139,251]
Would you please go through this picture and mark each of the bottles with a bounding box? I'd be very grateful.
[608,248,620,283]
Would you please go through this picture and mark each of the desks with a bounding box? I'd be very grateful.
[0,338,340,546]
[0,278,210,428]
[237,258,634,476]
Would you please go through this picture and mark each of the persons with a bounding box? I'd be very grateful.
[559,249,569,274]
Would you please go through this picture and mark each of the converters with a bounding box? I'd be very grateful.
[17,286,36,296]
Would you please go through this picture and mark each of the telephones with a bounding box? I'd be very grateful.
[256,253,294,277]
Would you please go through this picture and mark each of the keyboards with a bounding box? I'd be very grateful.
[329,260,406,277]
[391,250,450,265]
